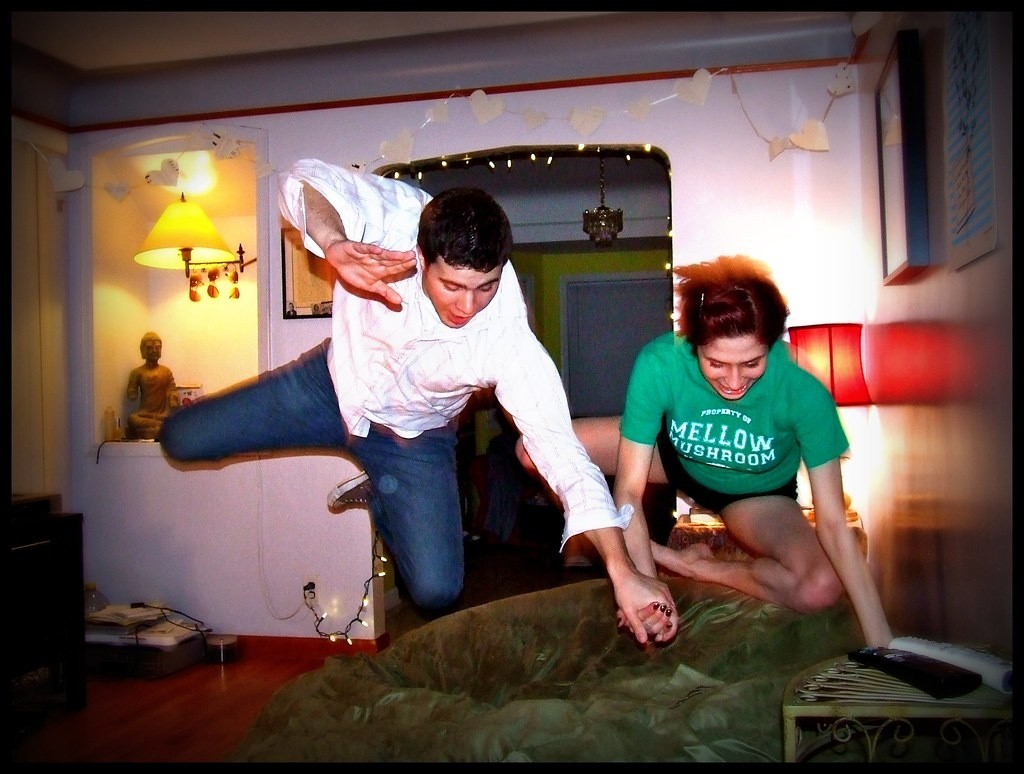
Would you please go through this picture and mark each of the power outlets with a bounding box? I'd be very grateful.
[301,574,319,609]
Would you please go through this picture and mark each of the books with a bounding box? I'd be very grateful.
[87,603,194,641]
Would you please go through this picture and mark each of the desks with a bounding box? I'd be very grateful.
[782,642,1015,763]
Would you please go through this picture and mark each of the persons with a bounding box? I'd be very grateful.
[161,160,678,643]
[127,331,180,441]
[516,255,893,650]
[286,303,297,314]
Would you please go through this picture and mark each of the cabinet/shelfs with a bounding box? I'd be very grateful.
[10,490,90,738]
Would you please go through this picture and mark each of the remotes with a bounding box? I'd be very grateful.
[846,635,1016,701]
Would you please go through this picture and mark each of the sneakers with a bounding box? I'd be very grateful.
[326,472,379,514]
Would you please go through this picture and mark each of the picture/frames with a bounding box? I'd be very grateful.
[876,29,929,285]
[281,227,333,318]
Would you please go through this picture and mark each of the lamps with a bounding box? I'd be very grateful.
[787,322,872,408]
[581,157,623,242]
[134,190,257,277]
